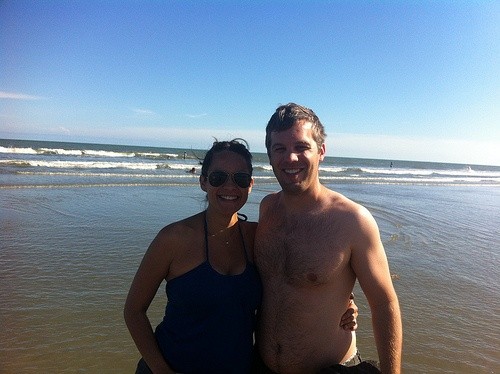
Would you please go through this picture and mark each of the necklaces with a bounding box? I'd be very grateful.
[209,223,239,248]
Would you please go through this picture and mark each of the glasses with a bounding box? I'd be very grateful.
[206,171,253,189]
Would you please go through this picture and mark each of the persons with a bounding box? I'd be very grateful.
[251,101,402,374]
[123,136,359,374]
